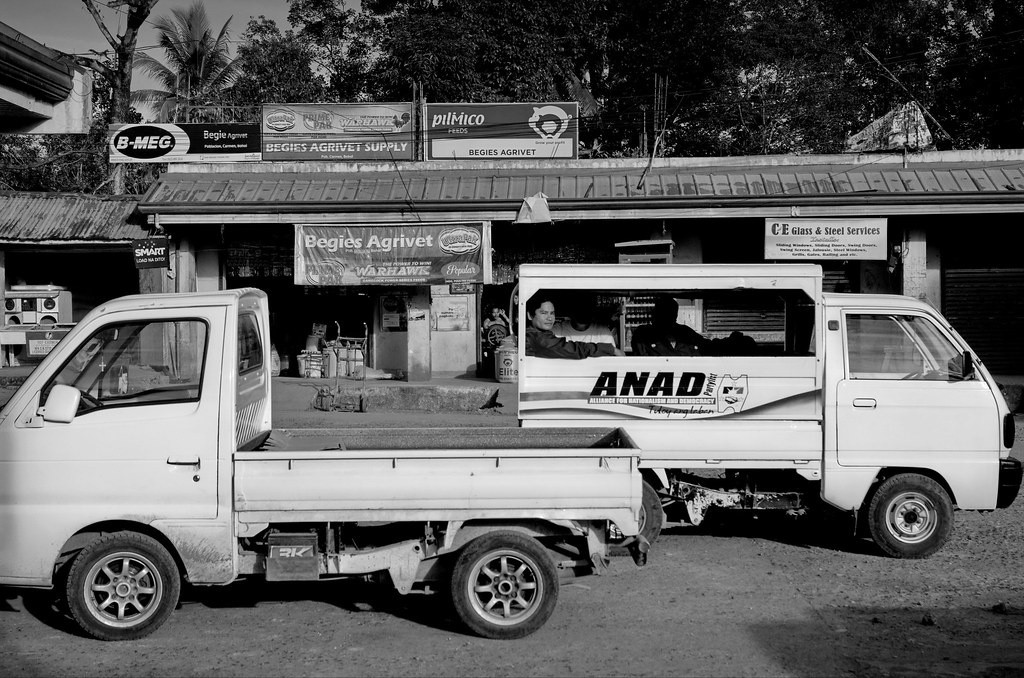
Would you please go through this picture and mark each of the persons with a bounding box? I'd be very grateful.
[483,305,508,329]
[526,294,626,360]
[633,300,744,356]
[499,291,519,338]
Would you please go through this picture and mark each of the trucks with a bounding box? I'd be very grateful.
[0,283,654,643]
[515,263,1024,560]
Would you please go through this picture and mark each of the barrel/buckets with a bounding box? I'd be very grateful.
[297,334,363,377]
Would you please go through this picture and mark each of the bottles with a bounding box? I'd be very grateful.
[627,330,633,347]
[626,297,655,324]
